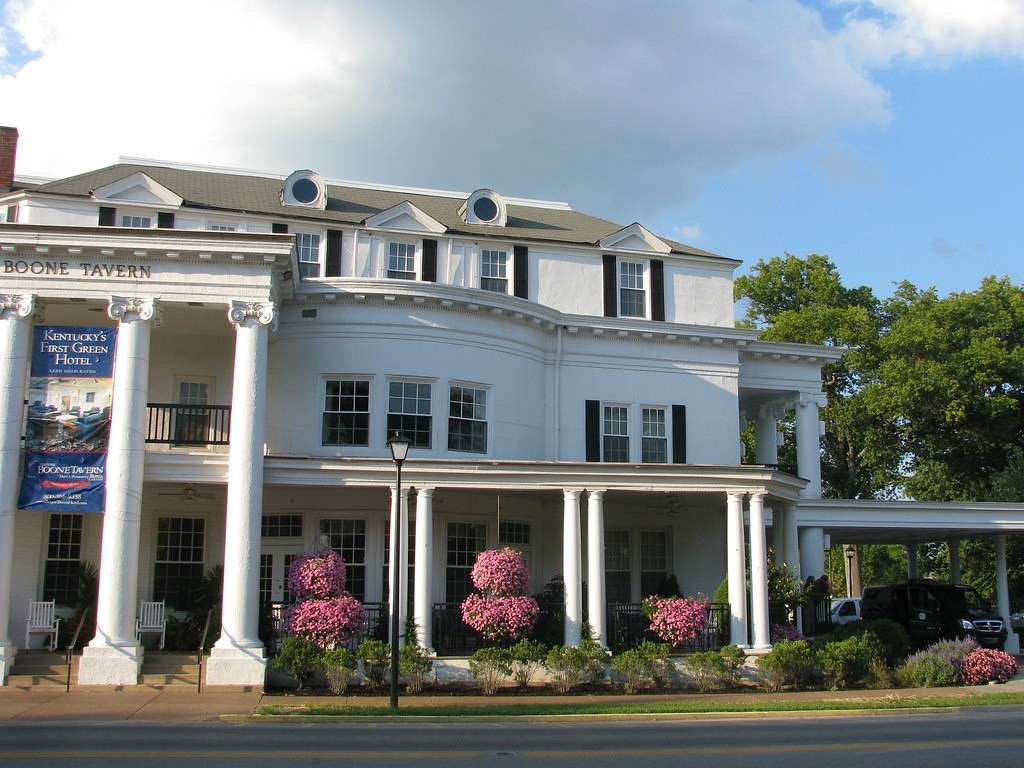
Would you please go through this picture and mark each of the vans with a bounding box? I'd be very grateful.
[859,578,1008,650]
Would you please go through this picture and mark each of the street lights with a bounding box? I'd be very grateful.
[845,545,856,599]
[385,429,411,710]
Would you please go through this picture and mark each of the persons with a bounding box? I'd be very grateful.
[812,575,829,607]
[804,575,815,590]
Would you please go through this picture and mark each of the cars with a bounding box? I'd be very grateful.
[786,597,862,629]
[1009,608,1024,643]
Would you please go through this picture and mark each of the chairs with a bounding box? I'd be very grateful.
[24,599,61,652]
[136,597,167,649]
